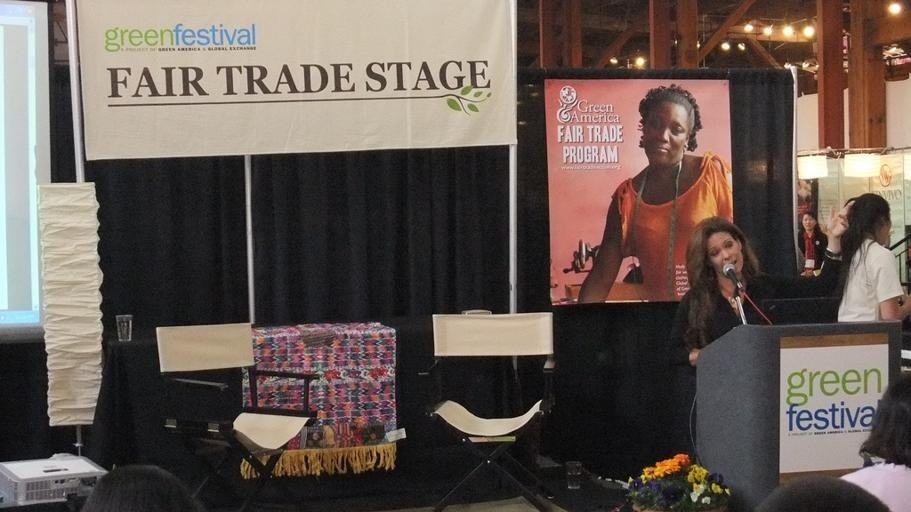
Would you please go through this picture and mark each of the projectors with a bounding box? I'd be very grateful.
[0,452,110,507]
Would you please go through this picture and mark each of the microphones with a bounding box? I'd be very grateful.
[723,263,745,293]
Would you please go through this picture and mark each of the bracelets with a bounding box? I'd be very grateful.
[823,248,842,262]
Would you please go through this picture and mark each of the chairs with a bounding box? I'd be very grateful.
[424,311,557,512]
[152,322,321,512]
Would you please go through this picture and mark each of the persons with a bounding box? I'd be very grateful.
[579,84,733,306]
[796,211,827,275]
[837,193,911,321]
[840,369,911,511]
[666,206,851,465]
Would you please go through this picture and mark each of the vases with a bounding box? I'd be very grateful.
[632,503,728,512]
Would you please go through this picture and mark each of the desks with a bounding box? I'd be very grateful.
[521,467,630,512]
[240,320,398,481]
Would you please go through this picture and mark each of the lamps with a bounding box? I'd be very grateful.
[797,152,911,181]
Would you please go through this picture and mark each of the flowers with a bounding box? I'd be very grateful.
[624,453,737,512]
